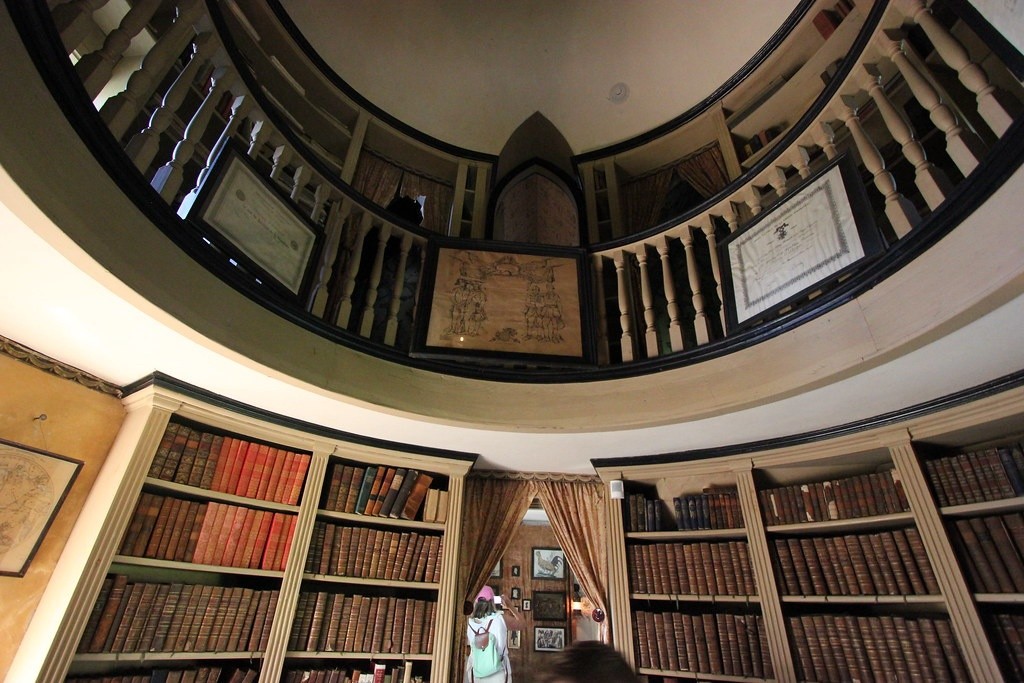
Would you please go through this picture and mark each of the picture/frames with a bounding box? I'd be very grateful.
[491,546,568,653]
[0,438,85,578]
[410,234,601,371]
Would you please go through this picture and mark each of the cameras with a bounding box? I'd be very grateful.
[494,597,503,604]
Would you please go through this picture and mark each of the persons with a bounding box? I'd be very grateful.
[466,585,525,683]
[534,640,640,683]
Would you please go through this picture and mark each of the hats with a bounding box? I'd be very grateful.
[474,584,495,602]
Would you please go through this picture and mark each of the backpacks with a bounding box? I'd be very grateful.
[468,620,506,677]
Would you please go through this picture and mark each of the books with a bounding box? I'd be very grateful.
[147,422,311,505]
[66,668,257,683]
[116,492,298,570]
[786,615,974,683]
[322,464,449,524]
[622,491,745,532]
[626,542,759,596]
[77,574,279,653]
[923,442,1024,507]
[757,469,911,526]
[304,521,445,582]
[768,527,940,596]
[947,513,1024,594]
[287,591,437,654]
[982,613,1024,683]
[283,661,430,683]
[631,610,775,683]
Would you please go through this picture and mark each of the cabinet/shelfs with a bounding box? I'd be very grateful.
[589,368,1024,683]
[0,371,480,683]
